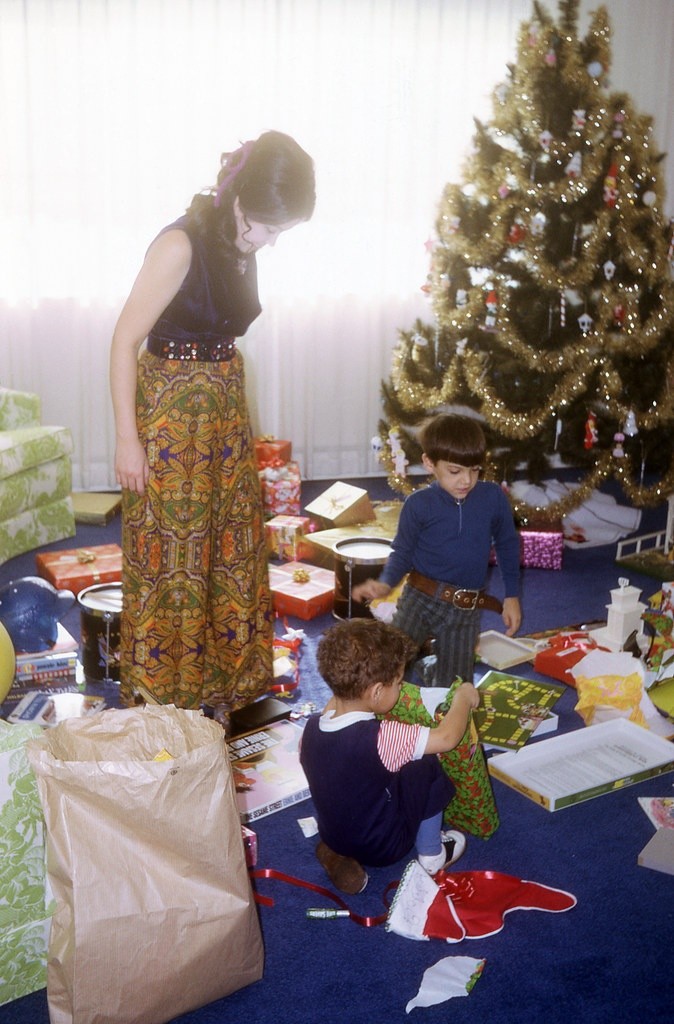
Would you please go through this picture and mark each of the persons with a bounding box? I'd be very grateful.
[298,618,481,897]
[108,129,315,710]
[352,413,522,688]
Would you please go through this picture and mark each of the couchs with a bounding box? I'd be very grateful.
[0,387,76,566]
[0,720,49,1006]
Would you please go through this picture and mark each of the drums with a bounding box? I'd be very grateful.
[331,536,395,622]
[77,581,124,685]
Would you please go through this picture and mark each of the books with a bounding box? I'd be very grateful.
[227,720,313,824]
[7,622,106,729]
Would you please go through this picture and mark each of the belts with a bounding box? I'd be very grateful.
[406,571,502,615]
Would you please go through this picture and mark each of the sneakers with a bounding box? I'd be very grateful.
[419,830,465,874]
[315,840,368,894]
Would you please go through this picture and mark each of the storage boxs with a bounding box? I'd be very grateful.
[537,642,611,688]
[225,719,312,823]
[241,825,258,866]
[488,717,674,812]
[75,493,123,526]
[480,711,558,751]
[252,437,564,620]
[36,542,124,596]
